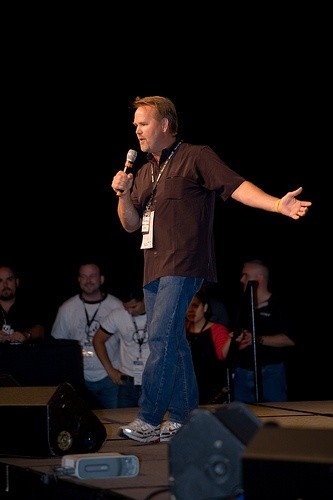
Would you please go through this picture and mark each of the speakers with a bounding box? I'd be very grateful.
[0,383,107,459]
[169,402,333,500]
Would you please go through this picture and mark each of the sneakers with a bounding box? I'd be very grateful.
[118,418,160,443]
[159,420,183,442]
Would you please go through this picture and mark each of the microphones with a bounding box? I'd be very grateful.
[117,149,137,196]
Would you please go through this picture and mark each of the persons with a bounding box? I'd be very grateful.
[112,96,312,443]
[0,259,304,409]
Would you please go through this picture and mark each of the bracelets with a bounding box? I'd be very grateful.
[26,332,33,338]
[273,199,281,213]
[258,335,263,345]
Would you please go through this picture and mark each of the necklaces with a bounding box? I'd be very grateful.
[187,320,208,332]
